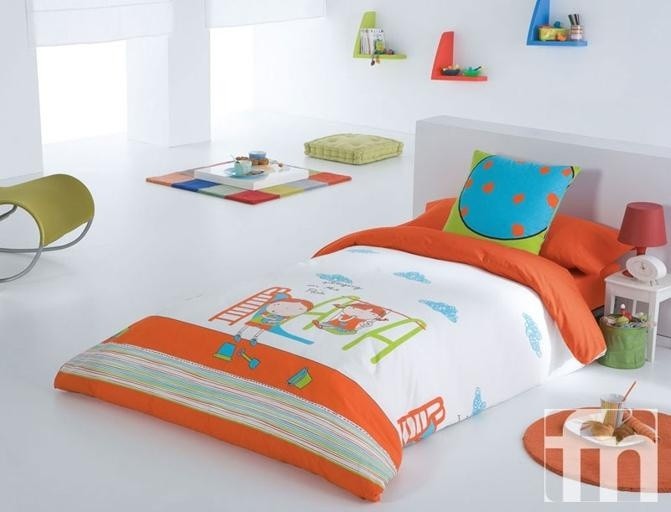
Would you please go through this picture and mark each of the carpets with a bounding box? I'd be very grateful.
[520,404,670,492]
[145,154,351,204]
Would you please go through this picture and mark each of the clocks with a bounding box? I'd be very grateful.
[625,253,666,287]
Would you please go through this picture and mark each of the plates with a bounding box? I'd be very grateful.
[564,408,651,447]
[223,166,272,177]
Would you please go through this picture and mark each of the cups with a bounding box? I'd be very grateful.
[600,394,632,431]
[232,151,269,175]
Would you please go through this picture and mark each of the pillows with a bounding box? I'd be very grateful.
[443,151,584,256]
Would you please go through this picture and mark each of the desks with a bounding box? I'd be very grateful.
[603,271,671,363]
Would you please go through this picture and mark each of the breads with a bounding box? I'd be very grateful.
[580,410,659,443]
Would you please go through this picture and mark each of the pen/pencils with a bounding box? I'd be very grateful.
[568,14,580,26]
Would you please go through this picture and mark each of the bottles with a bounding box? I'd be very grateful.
[569,24,583,42]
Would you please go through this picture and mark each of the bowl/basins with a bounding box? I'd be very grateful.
[444,69,479,77]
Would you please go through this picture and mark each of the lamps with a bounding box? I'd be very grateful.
[619,200,666,280]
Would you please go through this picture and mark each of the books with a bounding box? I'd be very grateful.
[359,27,386,54]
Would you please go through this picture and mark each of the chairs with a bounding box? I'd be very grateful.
[2,173,96,283]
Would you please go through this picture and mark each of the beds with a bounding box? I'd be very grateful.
[52,199,635,501]
